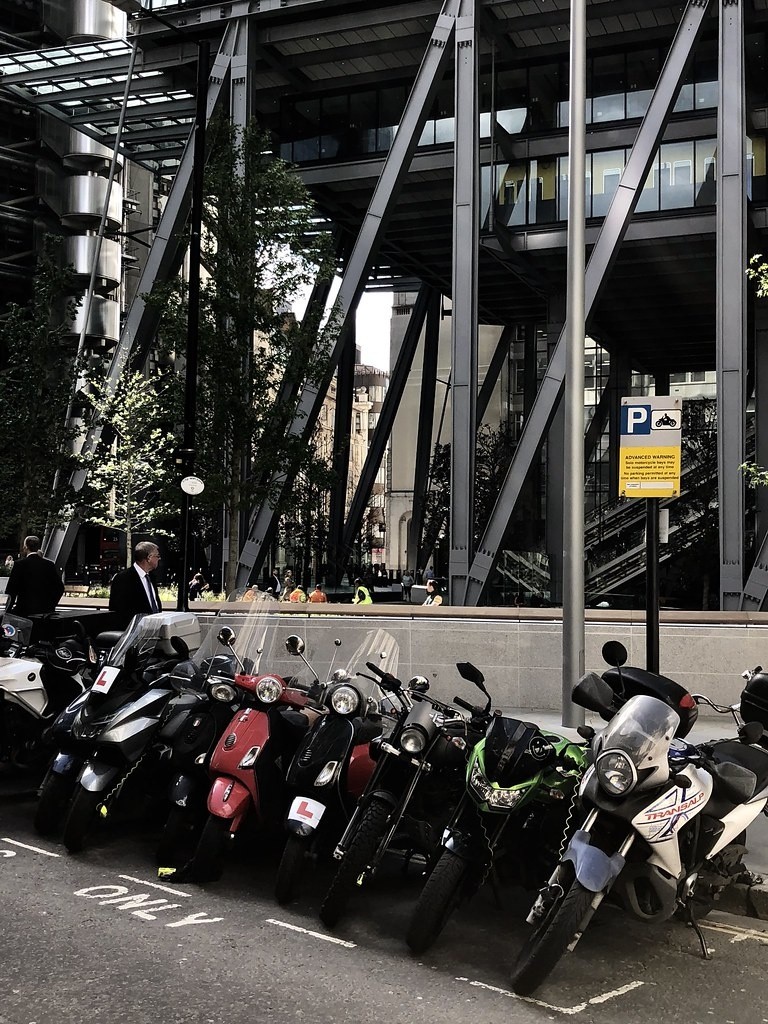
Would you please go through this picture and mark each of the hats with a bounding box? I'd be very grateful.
[266,587,273,594]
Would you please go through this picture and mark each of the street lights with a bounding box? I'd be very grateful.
[180,475,205,612]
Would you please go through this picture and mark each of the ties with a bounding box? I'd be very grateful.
[145,574,158,613]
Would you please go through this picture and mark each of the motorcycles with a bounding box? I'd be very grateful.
[508,639,767,997]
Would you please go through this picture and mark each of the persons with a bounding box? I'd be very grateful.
[239,569,310,603]
[4,535,65,614]
[422,578,443,606]
[108,541,162,613]
[188,573,209,601]
[351,576,374,605]
[400,565,434,602]
[310,583,328,602]
[346,562,389,592]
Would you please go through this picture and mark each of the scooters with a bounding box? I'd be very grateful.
[0,585,593,957]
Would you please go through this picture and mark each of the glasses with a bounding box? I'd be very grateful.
[146,553,161,560]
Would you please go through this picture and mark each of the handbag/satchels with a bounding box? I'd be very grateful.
[194,592,204,601]
